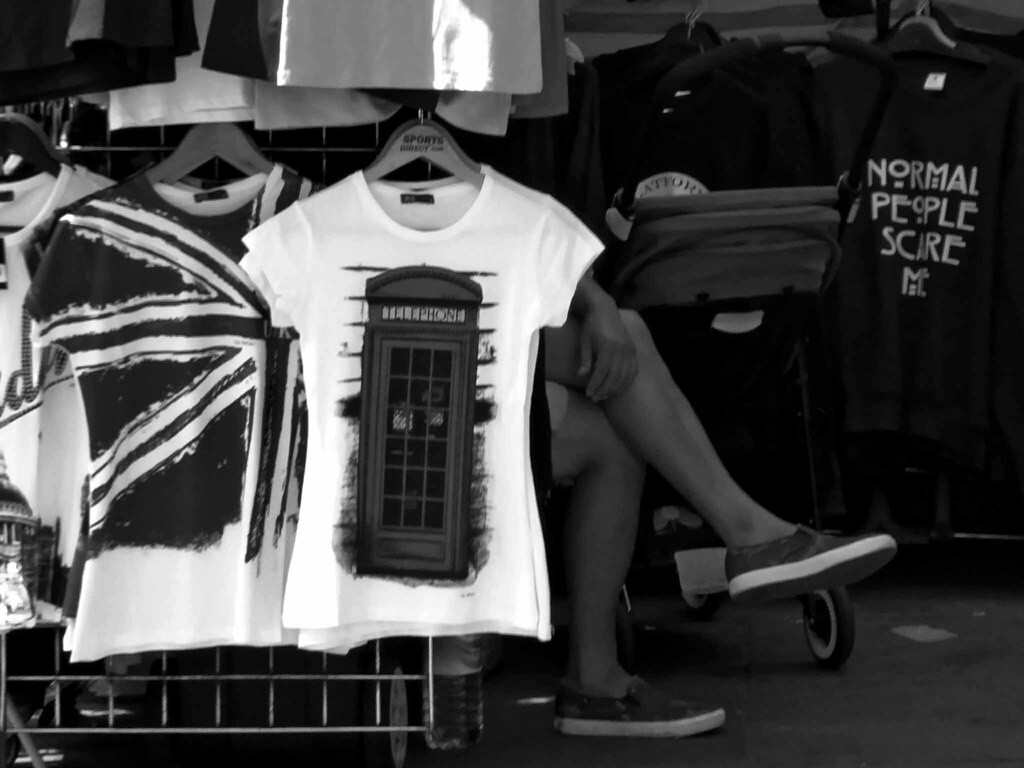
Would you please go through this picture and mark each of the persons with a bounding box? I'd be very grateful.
[528,275,897,736]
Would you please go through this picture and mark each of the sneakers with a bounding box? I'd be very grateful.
[552,675,726,739]
[725,524,897,602]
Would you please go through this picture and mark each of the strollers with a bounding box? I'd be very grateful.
[552,30,899,672]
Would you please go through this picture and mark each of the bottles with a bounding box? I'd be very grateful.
[421,638,486,750]
